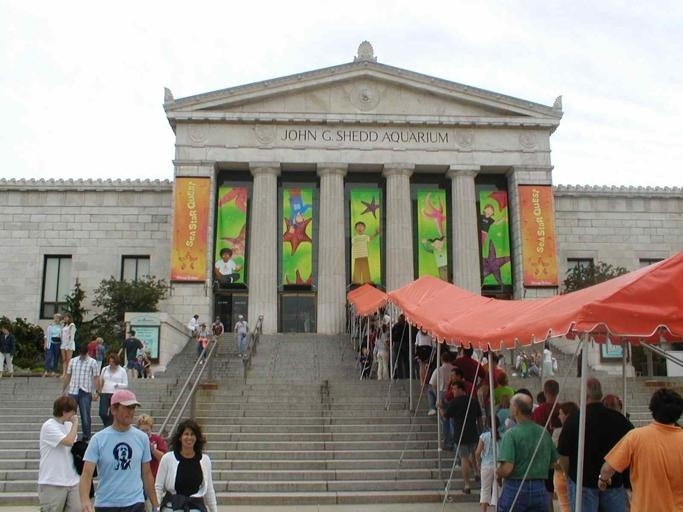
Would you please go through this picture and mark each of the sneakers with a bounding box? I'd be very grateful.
[428,410,435,415]
[238,354,247,357]
[214,353,218,358]
[199,358,206,365]
[463,488,471,494]
[130,375,154,379]
[43,371,65,379]
[475,476,481,481]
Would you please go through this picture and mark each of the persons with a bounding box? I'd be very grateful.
[303,308,313,333]
[233,315,250,356]
[0,324,16,379]
[38,313,224,512]
[597,388,683,512]
[350,306,637,511]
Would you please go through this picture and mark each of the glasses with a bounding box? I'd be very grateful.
[141,429,152,435]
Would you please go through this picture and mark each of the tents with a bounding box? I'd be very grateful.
[389,254,682,512]
[347,285,388,352]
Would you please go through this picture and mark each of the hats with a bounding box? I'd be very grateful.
[111,390,142,407]
[127,330,136,334]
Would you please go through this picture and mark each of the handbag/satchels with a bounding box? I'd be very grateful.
[52,337,60,342]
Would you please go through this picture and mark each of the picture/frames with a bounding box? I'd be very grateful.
[129,324,160,363]
[599,339,630,363]
